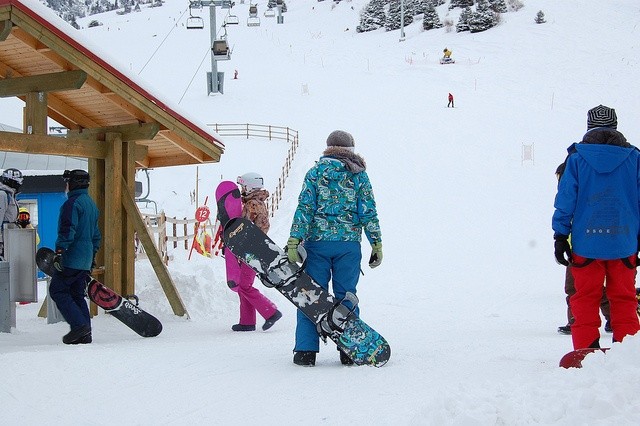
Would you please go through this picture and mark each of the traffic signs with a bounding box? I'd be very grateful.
[195,206,210,222]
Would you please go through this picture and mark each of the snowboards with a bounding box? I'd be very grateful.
[223,217,391,368]
[559,348,610,369]
[36,247,162,338]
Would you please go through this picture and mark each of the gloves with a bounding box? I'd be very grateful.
[53,250,64,273]
[369,242,383,269]
[554,233,572,266]
[287,238,301,263]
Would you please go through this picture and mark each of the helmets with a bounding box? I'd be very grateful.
[17,207,30,224]
[240,173,263,195]
[2,168,24,185]
[69,170,90,190]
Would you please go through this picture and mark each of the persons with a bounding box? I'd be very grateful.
[284,130,383,367]
[231,171,283,331]
[0,167,24,262]
[447,93,454,107]
[442,48,451,58]
[17,206,40,245]
[48,168,102,344]
[554,162,613,334]
[551,104,640,351]
[234,69,238,79]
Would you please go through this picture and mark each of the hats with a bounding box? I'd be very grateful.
[326,130,355,153]
[587,104,616,131]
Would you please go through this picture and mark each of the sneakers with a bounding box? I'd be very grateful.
[341,351,354,366]
[293,351,316,367]
[63,322,91,343]
[558,324,571,335]
[605,321,612,333]
[232,324,255,331]
[68,333,92,344]
[263,310,282,331]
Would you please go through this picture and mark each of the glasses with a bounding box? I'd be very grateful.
[18,213,29,220]
[237,177,262,188]
[63,170,88,179]
